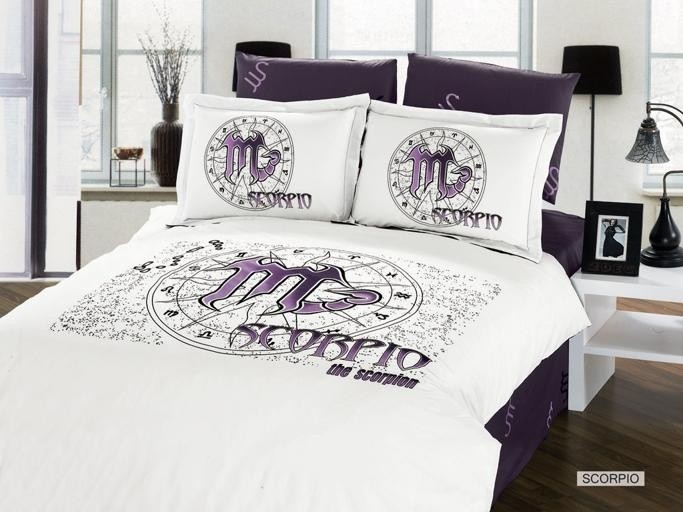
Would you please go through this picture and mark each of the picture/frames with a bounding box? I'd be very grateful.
[582,201,644,275]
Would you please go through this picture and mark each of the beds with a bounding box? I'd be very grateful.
[0,208,590,512]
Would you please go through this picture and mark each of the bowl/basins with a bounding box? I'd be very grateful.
[113,148,143,159]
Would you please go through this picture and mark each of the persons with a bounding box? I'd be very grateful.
[602,217,625,258]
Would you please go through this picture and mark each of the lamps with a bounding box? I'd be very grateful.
[625,102,683,268]
[231,42,292,92]
[563,45,622,202]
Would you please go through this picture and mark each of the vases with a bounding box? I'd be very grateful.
[149,102,184,187]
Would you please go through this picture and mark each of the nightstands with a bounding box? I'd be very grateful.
[568,262,683,412]
[75,185,177,271]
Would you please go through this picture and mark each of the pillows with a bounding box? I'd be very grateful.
[166,94,372,229]
[404,53,581,204]
[349,100,564,266]
[235,50,398,103]
